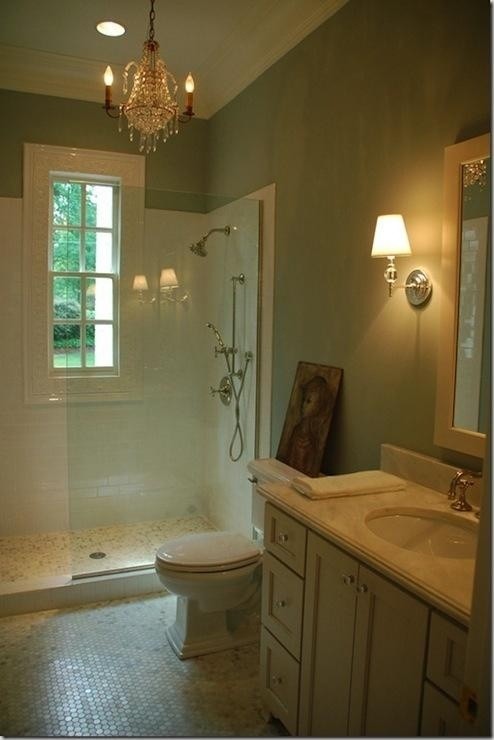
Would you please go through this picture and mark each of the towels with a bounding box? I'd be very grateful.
[290,469,408,501]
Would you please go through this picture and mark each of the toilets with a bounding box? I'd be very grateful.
[151,459,314,660]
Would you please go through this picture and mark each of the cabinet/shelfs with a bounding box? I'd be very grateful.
[255,500,474,737]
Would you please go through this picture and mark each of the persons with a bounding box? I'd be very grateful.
[282,375,330,477]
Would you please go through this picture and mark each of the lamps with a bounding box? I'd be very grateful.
[158,268,191,305]
[371,214,433,308]
[101,0,198,152]
[132,274,157,307]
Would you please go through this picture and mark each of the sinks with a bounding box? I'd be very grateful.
[365,506,479,560]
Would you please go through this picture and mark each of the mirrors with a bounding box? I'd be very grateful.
[433,132,492,458]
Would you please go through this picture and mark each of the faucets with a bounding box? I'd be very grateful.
[447,469,481,501]
